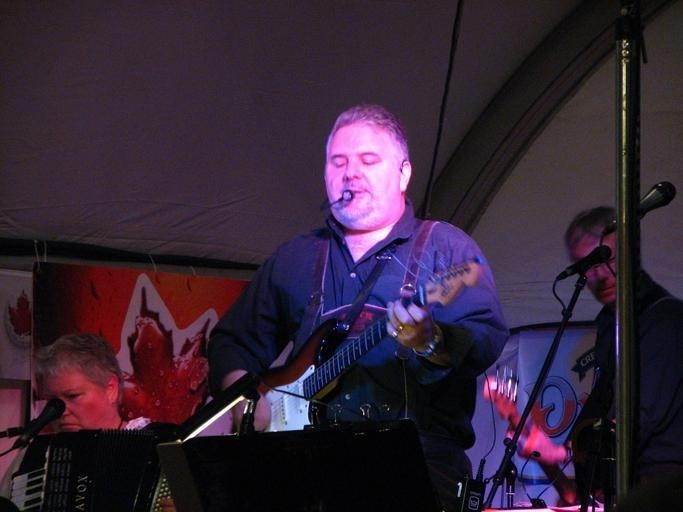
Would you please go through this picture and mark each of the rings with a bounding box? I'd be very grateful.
[390,331,398,338]
[396,324,404,332]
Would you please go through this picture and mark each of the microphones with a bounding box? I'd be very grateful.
[603,181,676,238]
[12,398,66,453]
[555,246,613,283]
[319,190,352,212]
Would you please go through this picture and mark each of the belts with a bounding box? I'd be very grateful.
[417,435,462,454]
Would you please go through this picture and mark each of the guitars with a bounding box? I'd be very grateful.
[257,255,487,432]
[484,364,605,512]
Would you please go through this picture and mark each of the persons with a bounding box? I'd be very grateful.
[504,205,681,510]
[0,331,178,512]
[205,103,511,512]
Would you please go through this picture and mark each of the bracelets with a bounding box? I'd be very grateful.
[562,443,573,465]
[410,321,443,359]
